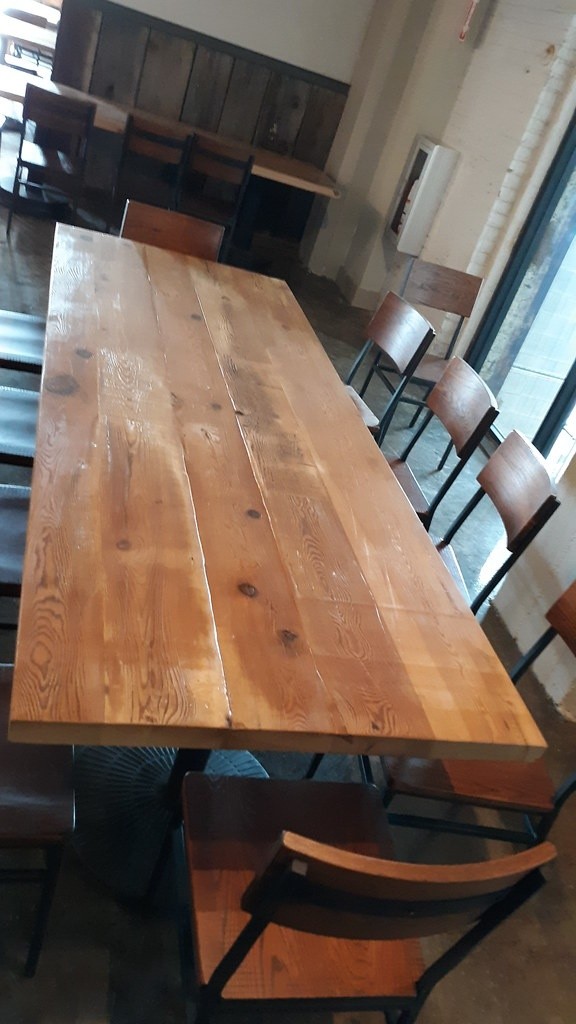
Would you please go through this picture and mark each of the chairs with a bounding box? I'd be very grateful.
[5,83,255,257]
[177,288,576,1024]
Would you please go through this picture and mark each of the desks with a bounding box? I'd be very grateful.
[6,220,547,916]
[0,64,344,200]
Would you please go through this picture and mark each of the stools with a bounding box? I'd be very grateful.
[0,385,39,468]
[0,483,31,665]
[0,310,47,375]
